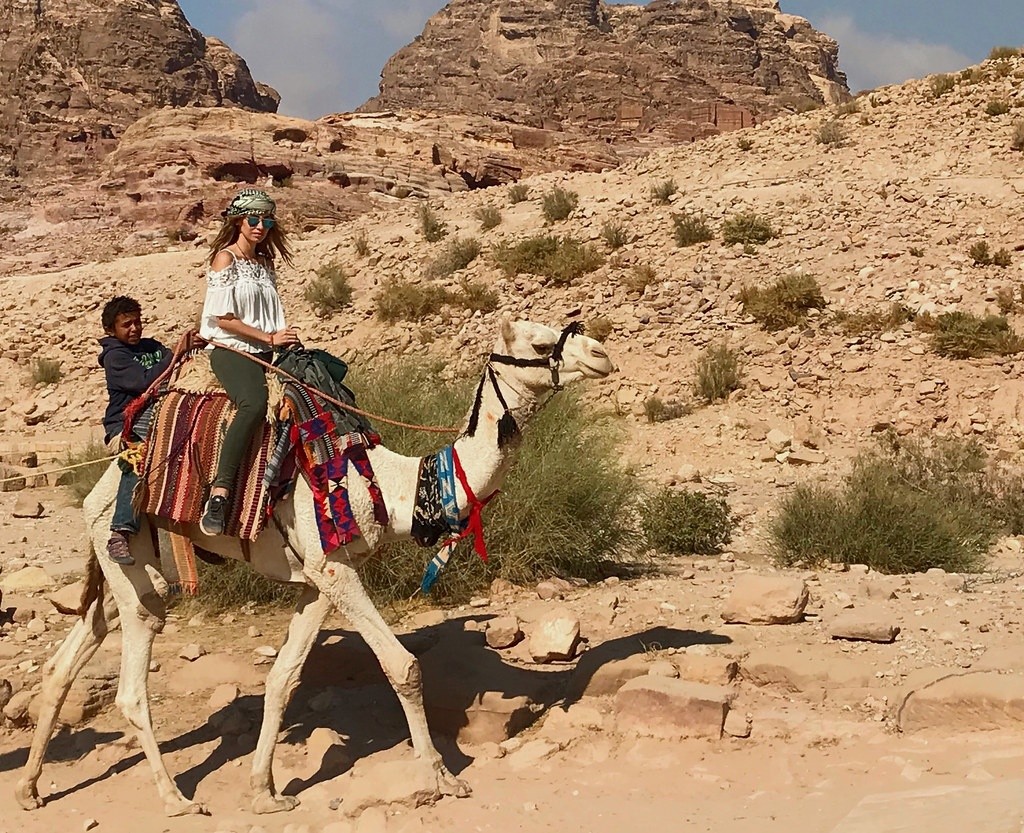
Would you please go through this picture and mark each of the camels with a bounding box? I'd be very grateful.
[13,312,613,816]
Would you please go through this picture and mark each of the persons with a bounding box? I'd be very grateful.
[95,295,209,564]
[197,187,308,536]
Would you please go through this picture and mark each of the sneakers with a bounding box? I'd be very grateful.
[199,493,229,536]
[106,538,135,565]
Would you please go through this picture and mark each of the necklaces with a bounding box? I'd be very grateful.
[235,240,261,279]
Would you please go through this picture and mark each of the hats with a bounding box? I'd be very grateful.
[226,189,276,218]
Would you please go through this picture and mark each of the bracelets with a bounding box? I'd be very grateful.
[271,334,274,348]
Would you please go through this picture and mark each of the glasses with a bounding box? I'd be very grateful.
[247,216,275,229]
